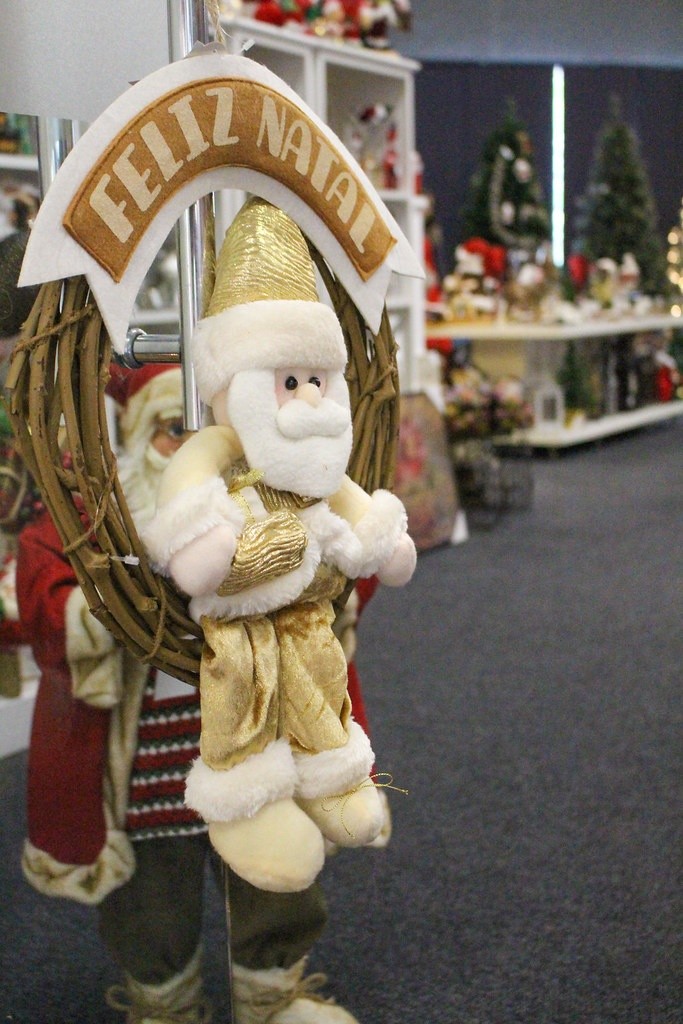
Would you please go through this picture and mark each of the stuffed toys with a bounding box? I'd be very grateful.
[148,196,418,891]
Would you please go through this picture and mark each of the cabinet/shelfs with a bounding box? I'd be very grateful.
[427,313,683,448]
[214,14,425,393]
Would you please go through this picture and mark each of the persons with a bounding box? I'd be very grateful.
[14,363,364,1024]
